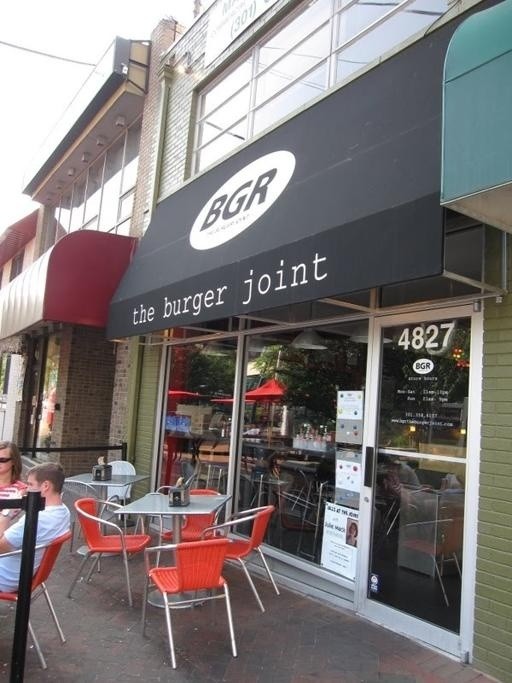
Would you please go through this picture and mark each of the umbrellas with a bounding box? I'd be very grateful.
[168,388,199,406]
[210,379,296,443]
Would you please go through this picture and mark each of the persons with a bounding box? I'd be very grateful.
[0,440,71,593]
[376,446,422,530]
[316,430,336,490]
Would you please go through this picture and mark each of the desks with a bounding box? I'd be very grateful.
[113,494,233,610]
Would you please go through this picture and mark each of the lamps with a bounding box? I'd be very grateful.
[289,302,327,351]
[346,288,391,344]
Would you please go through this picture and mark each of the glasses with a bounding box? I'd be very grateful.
[0,456,12,464]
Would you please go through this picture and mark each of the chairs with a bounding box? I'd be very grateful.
[402,517,464,604]
[192,504,280,614]
[1,529,75,668]
[143,538,238,670]
[66,498,151,606]
[63,458,223,573]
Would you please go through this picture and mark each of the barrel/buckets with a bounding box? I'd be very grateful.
[92,456,112,481]
[168,477,190,507]
